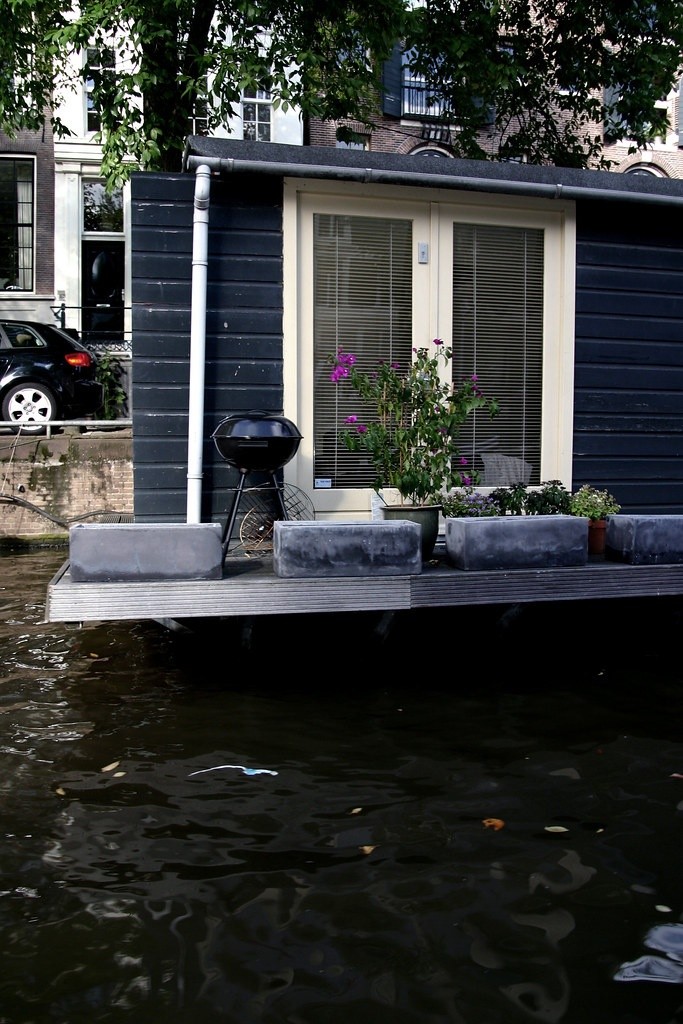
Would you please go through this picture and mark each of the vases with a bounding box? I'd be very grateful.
[381,505,443,561]
[445,514,589,570]
[604,513,683,565]
[589,520,607,553]
[69,522,223,582]
[273,520,423,578]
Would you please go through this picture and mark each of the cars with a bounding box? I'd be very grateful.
[0,317,113,437]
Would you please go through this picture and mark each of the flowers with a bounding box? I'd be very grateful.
[441,480,621,518]
[327,338,502,507]
